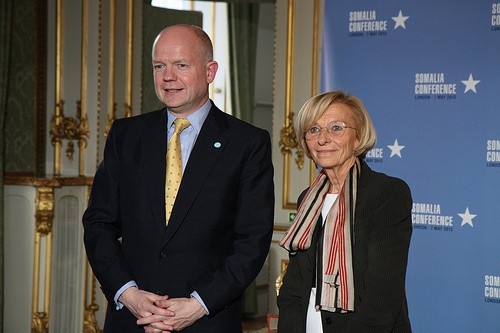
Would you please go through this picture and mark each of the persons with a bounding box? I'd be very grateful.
[83,24,274,333]
[276,90,413,333]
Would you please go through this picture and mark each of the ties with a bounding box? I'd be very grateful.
[165,117,191,227]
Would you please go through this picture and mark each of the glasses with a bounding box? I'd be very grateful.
[304,120,360,141]
